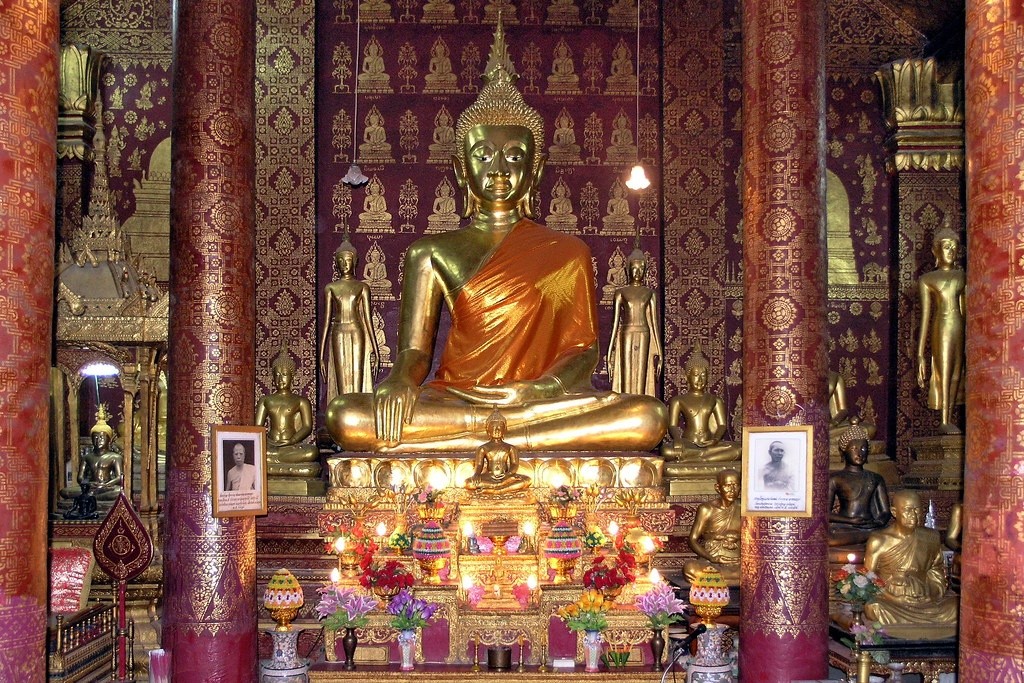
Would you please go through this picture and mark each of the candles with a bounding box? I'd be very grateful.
[474,635,478,646]
[519,635,524,645]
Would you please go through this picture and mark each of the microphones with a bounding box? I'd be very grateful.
[674,624,707,651]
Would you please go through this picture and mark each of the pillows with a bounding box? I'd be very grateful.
[49,546,95,612]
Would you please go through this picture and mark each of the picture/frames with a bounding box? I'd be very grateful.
[210,423,268,518]
[740,426,813,518]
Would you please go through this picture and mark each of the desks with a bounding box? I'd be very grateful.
[827,622,958,683]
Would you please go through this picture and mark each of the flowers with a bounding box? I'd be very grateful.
[323,523,377,554]
[361,553,415,589]
[556,590,614,630]
[412,483,443,509]
[584,525,606,546]
[582,551,637,589]
[635,582,683,625]
[831,563,884,603]
[386,588,439,629]
[317,583,378,630]
[550,485,580,508]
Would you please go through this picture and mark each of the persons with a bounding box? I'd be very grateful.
[227,443,256,491]
[606,249,664,397]
[60,419,123,500]
[120,370,167,448]
[826,369,875,442]
[662,335,742,463]
[762,440,793,490]
[256,352,318,462]
[827,425,893,548]
[323,10,669,451]
[318,241,380,406]
[463,404,532,493]
[682,469,741,583]
[944,503,962,551]
[916,221,967,432]
[864,490,960,623]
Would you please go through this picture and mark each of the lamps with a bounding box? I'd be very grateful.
[341,0,370,187]
[624,0,652,191]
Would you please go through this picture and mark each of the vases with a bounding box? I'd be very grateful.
[374,585,400,610]
[340,553,359,582]
[601,586,622,601]
[582,630,602,673]
[342,626,357,671]
[560,507,567,521]
[590,546,598,554]
[652,627,664,672]
[397,629,415,671]
[849,608,864,629]
[424,506,432,517]
